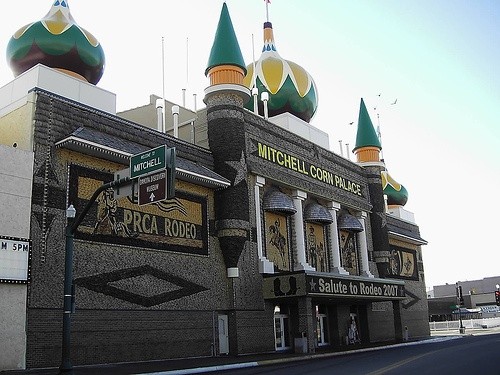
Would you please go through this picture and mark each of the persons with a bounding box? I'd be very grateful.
[273,220,282,245]
[307,225,318,270]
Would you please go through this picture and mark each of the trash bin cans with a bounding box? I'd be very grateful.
[402,325,409,341]
[294,332,308,354]
[459,328,465,333]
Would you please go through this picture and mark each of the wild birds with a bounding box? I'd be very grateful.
[390,98,397,105]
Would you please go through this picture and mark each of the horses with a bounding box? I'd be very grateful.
[266,225,287,267]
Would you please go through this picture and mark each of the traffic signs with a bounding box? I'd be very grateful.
[115,145,176,206]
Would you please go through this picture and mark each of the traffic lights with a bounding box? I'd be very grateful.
[495,290,500,306]
